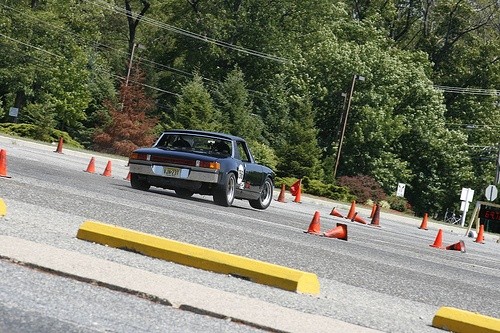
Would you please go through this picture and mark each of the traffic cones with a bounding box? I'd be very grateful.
[0,148,11,178]
[429,228,444,249]
[292,185,302,203]
[417,213,429,230]
[101,160,112,177]
[53,137,64,154]
[369,204,381,227]
[351,212,367,224]
[329,206,344,218]
[323,223,348,241]
[367,200,376,218]
[303,210,323,236]
[445,240,465,253]
[274,183,287,203]
[345,200,355,219]
[83,156,96,173]
[473,224,485,244]
[126,172,132,181]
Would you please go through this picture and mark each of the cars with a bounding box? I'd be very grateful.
[128,129,277,210]
